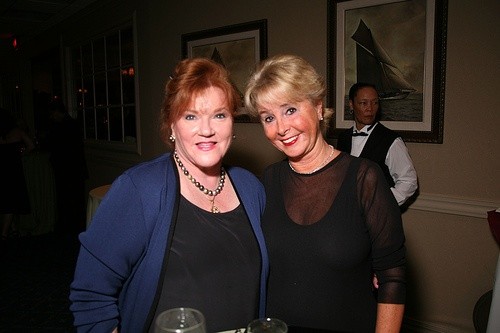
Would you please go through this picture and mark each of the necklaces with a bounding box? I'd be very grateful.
[175,149,227,213]
[288,144,334,175]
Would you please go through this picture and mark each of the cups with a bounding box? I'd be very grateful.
[247,318,288,333]
[154,308,207,333]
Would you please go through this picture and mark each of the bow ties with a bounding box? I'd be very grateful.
[352,122,377,137]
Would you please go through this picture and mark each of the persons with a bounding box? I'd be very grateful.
[244,54,410,333]
[68,57,270,333]
[0,105,35,245]
[35,99,90,246]
[329,81,418,210]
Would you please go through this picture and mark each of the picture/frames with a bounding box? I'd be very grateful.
[181,18,268,123]
[326,0,449,144]
